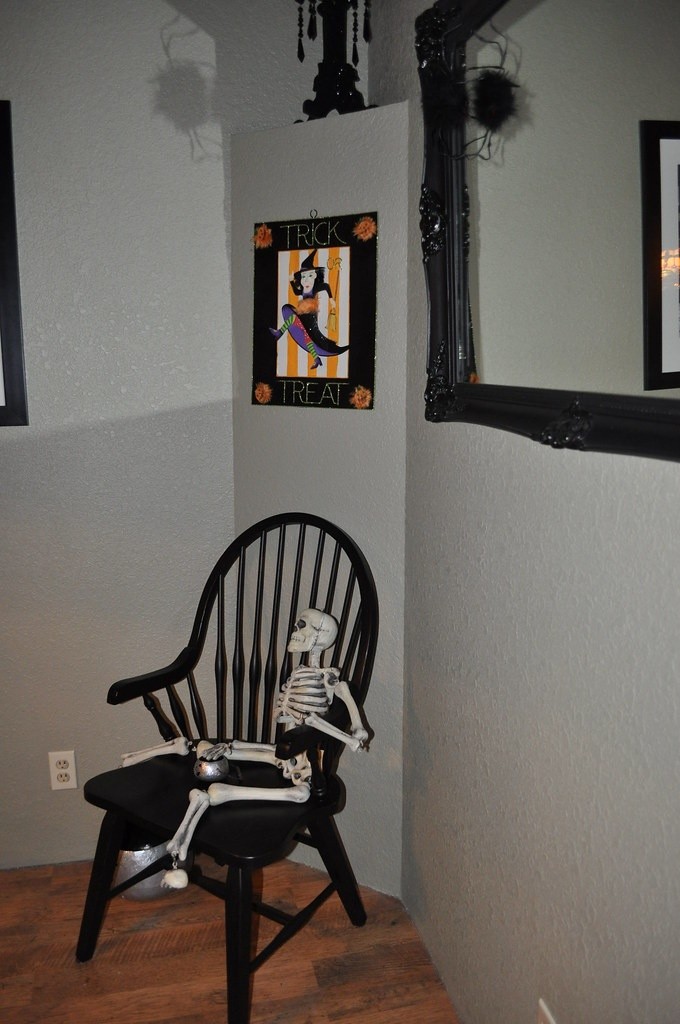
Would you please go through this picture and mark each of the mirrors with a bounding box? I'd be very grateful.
[414,1,679,462]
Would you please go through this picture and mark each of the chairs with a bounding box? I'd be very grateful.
[76,511,380,1024]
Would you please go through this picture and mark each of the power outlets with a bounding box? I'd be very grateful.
[49,750,78,792]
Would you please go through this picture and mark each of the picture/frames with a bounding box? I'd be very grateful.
[638,119,680,392]
[0,99,30,428]
